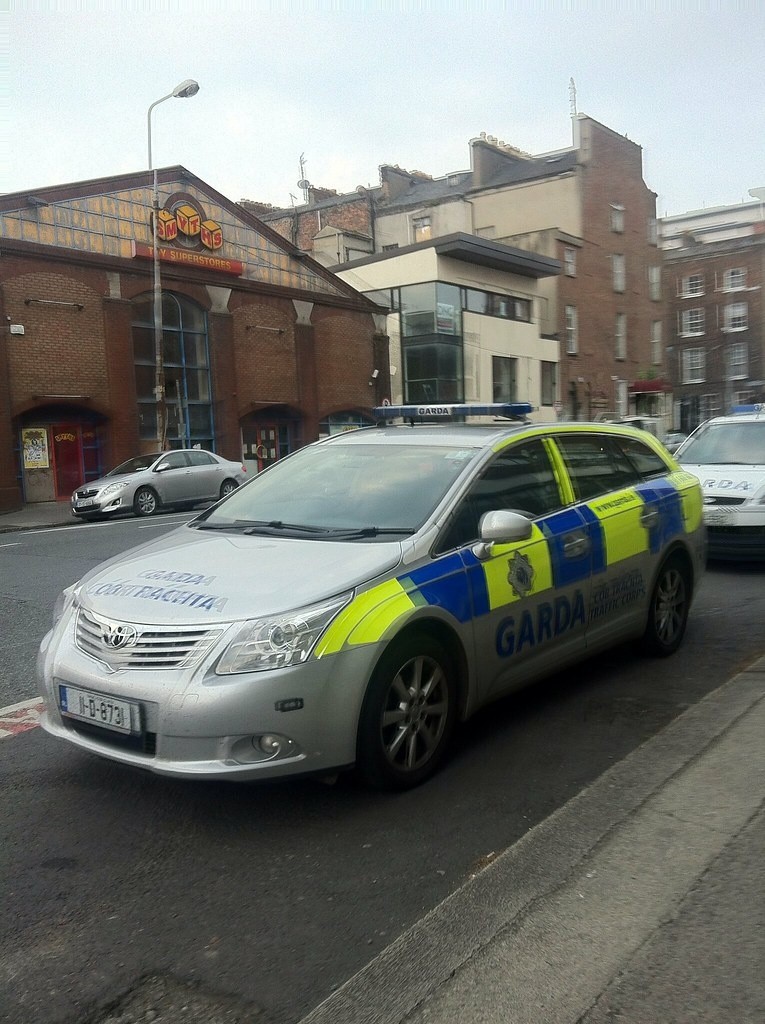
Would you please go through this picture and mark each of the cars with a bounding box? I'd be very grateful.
[71,448,247,521]
[38,403,707,794]
[674,402,765,554]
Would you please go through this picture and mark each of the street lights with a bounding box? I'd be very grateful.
[147,79,200,170]
